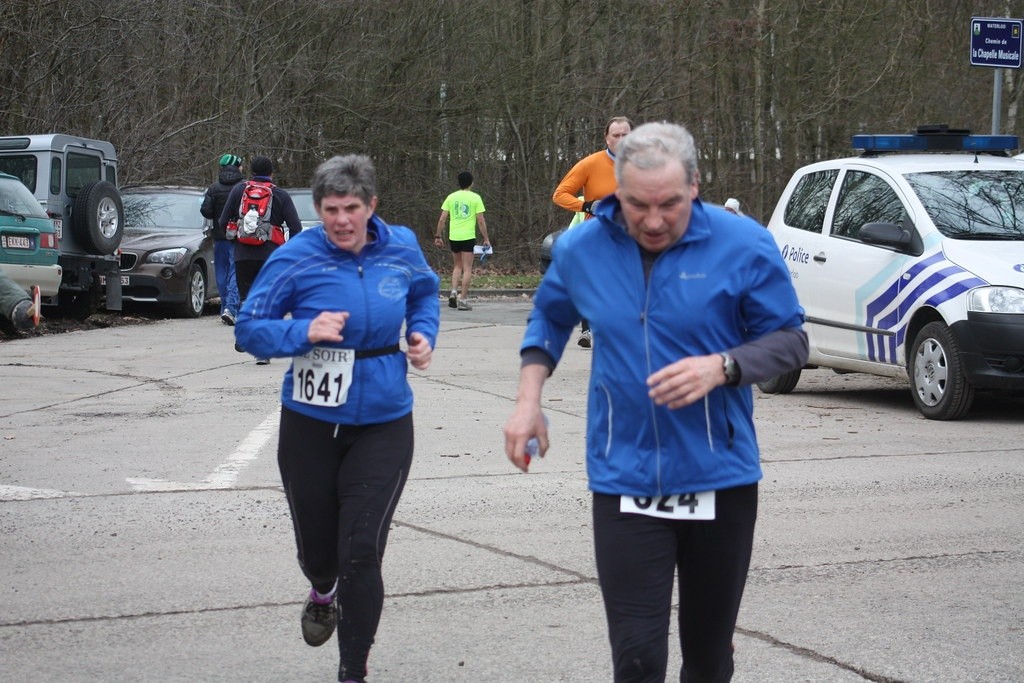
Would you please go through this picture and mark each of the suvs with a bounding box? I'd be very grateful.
[0,134,123,307]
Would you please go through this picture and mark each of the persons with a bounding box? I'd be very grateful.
[725,198,740,214]
[568,196,591,348]
[218,158,302,364]
[235,153,439,683]
[200,155,246,326]
[434,172,490,310]
[503,122,809,683]
[553,117,634,220]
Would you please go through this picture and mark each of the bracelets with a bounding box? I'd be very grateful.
[435,236,442,238]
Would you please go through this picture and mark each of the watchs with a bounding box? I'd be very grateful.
[721,352,740,384]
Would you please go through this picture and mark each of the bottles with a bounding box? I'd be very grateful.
[525,416,550,465]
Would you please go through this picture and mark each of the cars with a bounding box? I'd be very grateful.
[119,185,220,316]
[282,189,326,240]
[755,123,1024,421]
[540,227,563,272]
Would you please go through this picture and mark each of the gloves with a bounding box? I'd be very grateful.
[582,200,596,218]
[12,300,44,337]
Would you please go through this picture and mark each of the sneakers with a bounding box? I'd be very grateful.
[301,578,338,646]
[338,677,366,683]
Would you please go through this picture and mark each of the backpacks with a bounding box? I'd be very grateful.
[225,181,285,246]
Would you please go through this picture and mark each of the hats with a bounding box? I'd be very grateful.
[250,157,273,176]
[725,198,740,213]
[219,153,242,168]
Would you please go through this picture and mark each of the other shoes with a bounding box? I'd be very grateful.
[235,340,246,353]
[449,293,457,308]
[458,301,473,311]
[218,316,228,325]
[254,357,270,365]
[221,309,236,326]
[577,329,592,348]
